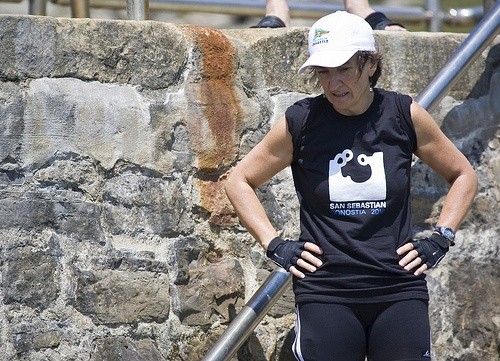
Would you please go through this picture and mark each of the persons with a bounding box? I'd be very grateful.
[249,0,411,32]
[221,7,479,360]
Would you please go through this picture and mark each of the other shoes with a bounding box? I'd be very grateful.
[255,16,286,28]
[364,12,405,31]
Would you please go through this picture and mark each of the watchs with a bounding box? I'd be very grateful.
[433,226,456,241]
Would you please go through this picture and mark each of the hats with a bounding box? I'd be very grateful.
[297,9,377,76]
